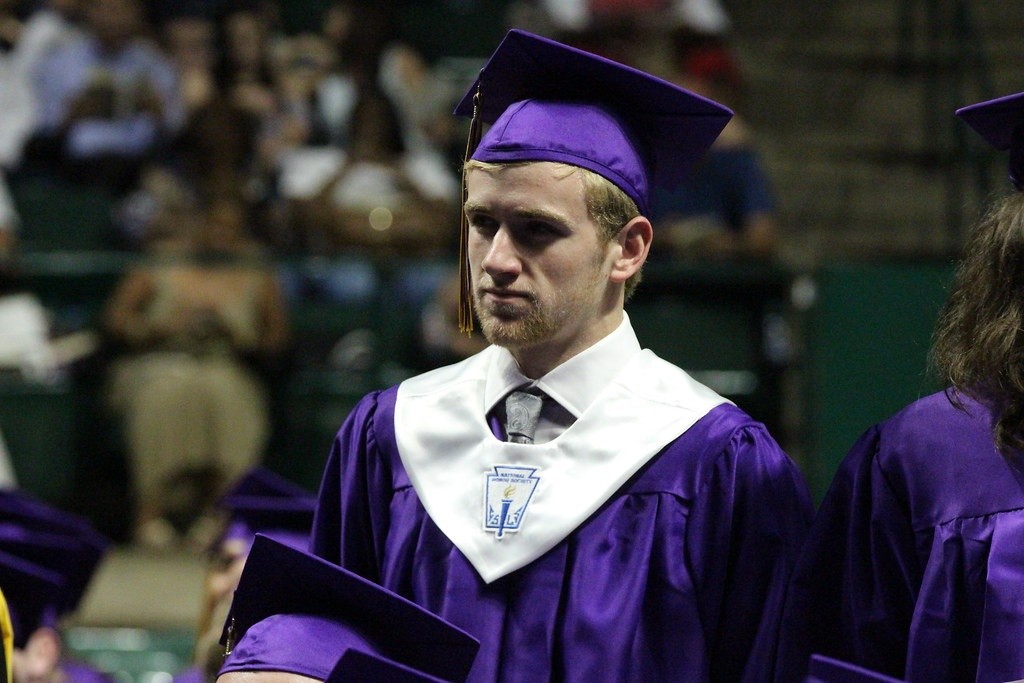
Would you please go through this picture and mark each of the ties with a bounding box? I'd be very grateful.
[497,390,552,446]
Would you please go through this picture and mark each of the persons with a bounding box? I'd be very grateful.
[0,0,1024,683]
[310,28,817,683]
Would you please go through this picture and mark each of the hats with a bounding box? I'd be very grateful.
[216,465,316,539]
[322,645,464,681]
[0,492,112,651]
[956,88,1024,177]
[214,531,480,680]
[451,25,736,340]
